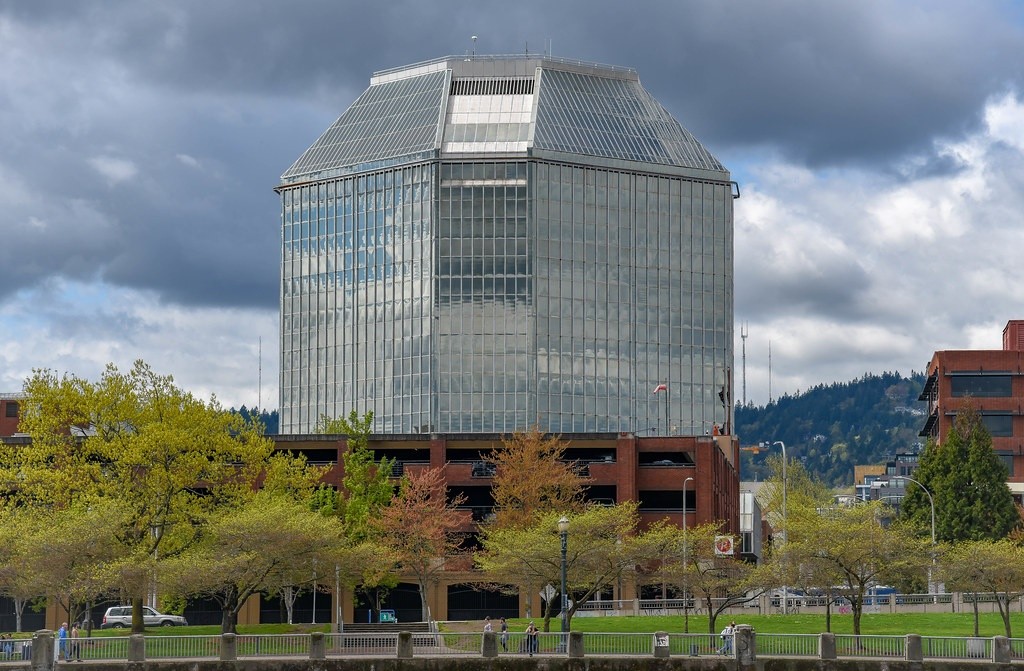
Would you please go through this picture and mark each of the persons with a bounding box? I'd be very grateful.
[525,620,539,658]
[5,633,15,660]
[57,622,72,661]
[715,624,735,656]
[483,616,492,633]
[0,633,6,653]
[69,622,83,664]
[497,617,511,654]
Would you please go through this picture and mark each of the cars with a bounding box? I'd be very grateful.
[745,585,805,607]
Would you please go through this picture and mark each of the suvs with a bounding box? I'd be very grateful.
[101,604,188,629]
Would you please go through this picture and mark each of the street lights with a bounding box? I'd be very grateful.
[557,516,570,653]
[891,474,937,606]
[773,440,787,615]
[682,477,695,633]
[833,494,905,606]
